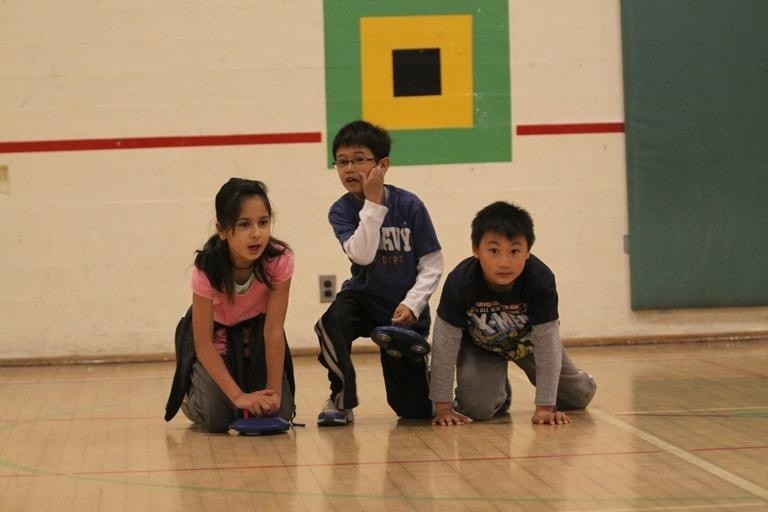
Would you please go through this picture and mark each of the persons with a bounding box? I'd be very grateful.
[314,120,444,427]
[165,179,294,430]
[431,201,597,426]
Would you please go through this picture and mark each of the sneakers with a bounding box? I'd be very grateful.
[317,398,354,425]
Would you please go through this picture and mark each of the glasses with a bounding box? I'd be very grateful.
[331,157,378,169]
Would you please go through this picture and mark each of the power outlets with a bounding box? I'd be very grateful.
[320,275,336,303]
